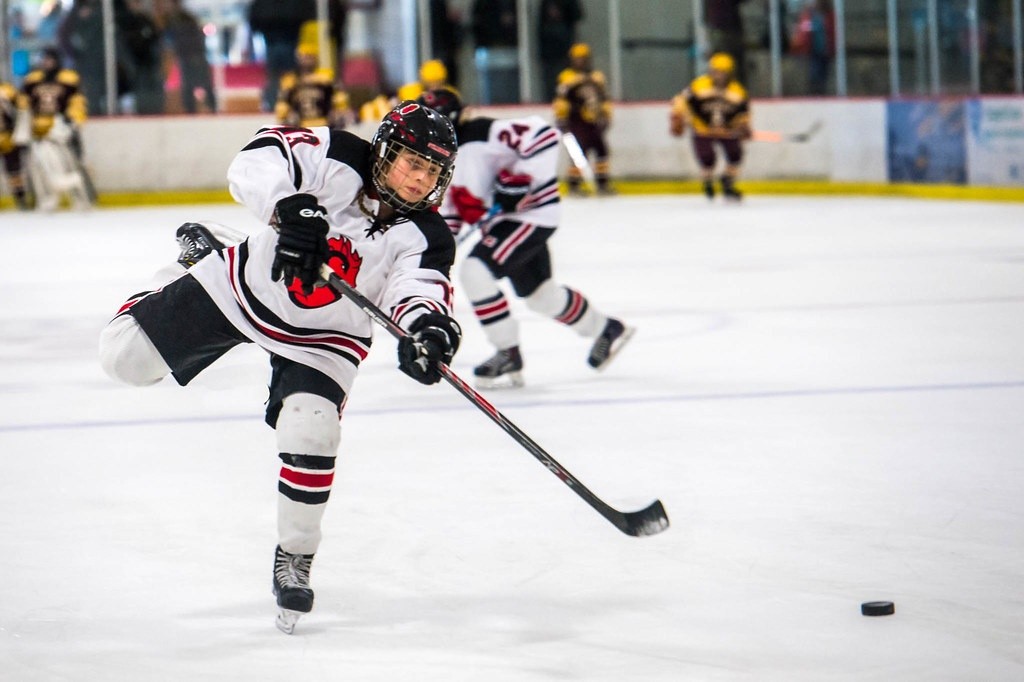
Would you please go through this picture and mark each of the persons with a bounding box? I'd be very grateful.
[0,46,98,212]
[551,44,618,196]
[358,59,460,125]
[673,51,753,207]
[415,85,636,390]
[9,0,838,116]
[273,44,351,126]
[96,101,462,634]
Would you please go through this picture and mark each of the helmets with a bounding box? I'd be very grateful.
[370,99,459,217]
[708,52,733,88]
[420,59,448,81]
[296,44,321,72]
[399,82,422,100]
[570,44,593,72]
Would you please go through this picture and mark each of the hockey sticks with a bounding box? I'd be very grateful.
[317,264,669,538]
[559,129,601,191]
[696,119,825,144]
[27,140,92,212]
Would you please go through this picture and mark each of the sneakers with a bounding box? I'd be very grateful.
[474,346,524,391]
[596,183,620,201]
[174,220,248,269]
[701,177,717,206]
[272,544,314,635]
[566,175,590,199]
[587,317,635,371]
[721,175,744,206]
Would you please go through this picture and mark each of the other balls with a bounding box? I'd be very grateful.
[862,600,895,617]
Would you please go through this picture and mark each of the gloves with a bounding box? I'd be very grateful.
[399,310,462,385]
[271,194,330,295]
